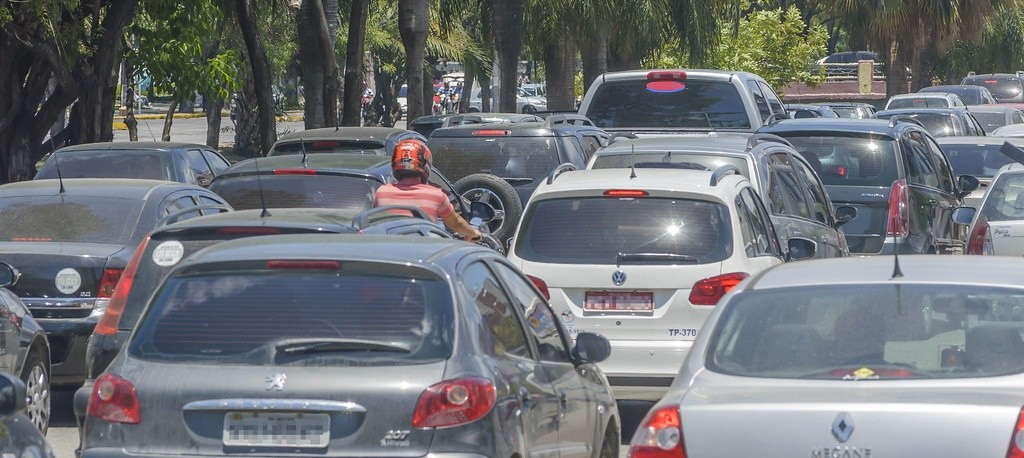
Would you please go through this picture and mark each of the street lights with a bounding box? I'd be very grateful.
[365,50,382,75]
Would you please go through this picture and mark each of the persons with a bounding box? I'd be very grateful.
[362,82,374,115]
[434,80,466,117]
[372,138,481,248]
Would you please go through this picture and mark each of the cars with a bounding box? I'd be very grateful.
[230,85,287,118]
[0,371,56,458]
[625,254,1024,457]
[586,131,859,263]
[382,83,408,120]
[79,235,621,458]
[813,51,913,81]
[783,104,839,119]
[885,92,966,109]
[917,86,998,105]
[410,112,545,138]
[868,108,986,139]
[811,102,877,120]
[0,178,237,415]
[932,135,1024,229]
[573,68,789,130]
[206,154,468,222]
[506,163,789,408]
[267,126,429,157]
[963,104,1023,135]
[32,142,231,189]
[0,261,52,439]
[993,123,1024,138]
[73,208,456,458]
[452,84,548,114]
[951,162,1024,257]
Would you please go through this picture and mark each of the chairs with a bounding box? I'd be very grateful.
[965,323,1024,376]
[832,310,886,365]
[770,324,828,375]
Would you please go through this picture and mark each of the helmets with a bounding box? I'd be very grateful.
[457,81,462,89]
[444,81,450,86]
[393,140,433,183]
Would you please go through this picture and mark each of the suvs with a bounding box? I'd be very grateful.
[957,71,1024,105]
[755,114,981,256]
[425,123,596,256]
